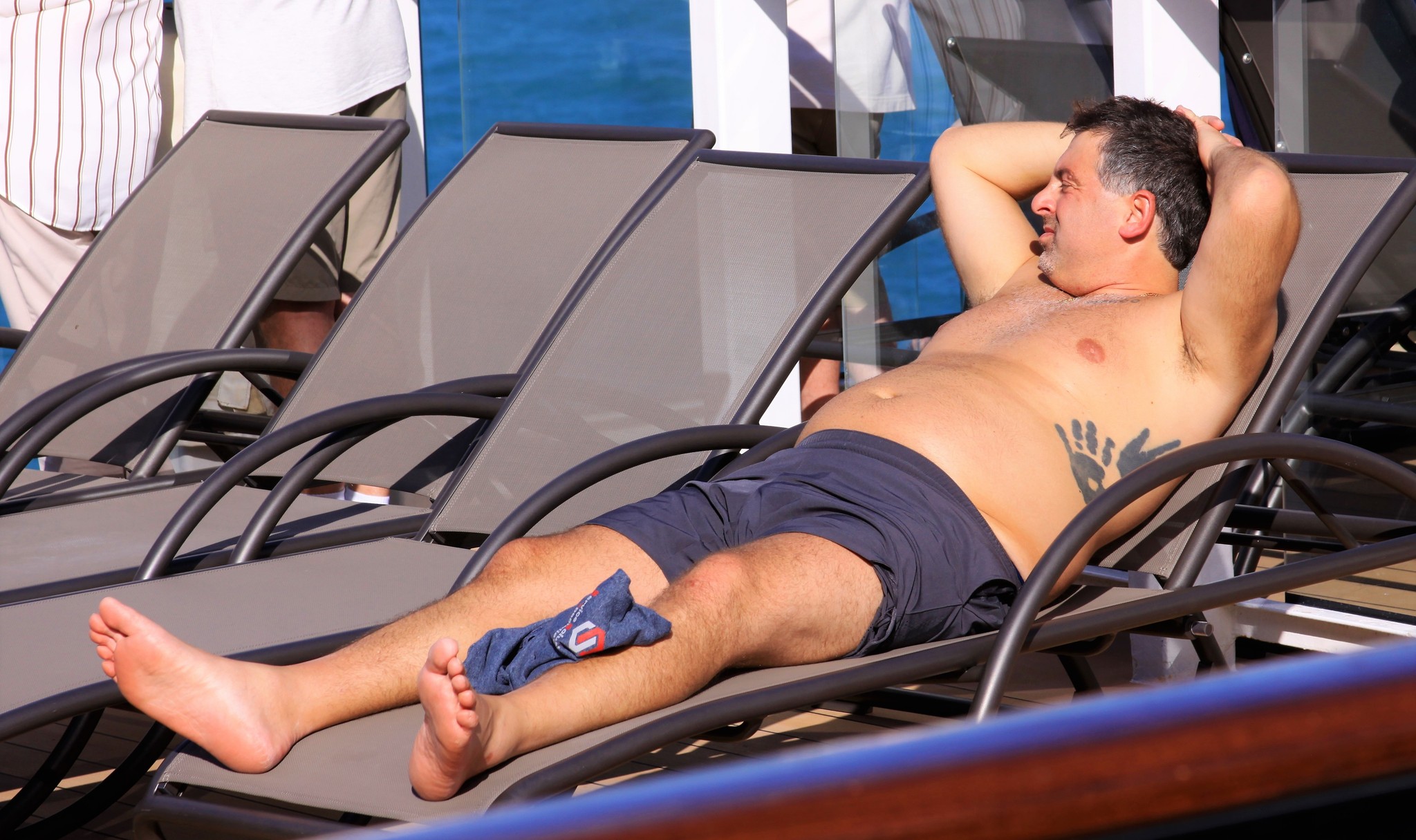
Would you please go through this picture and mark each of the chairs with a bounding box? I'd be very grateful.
[0,107,1416,840]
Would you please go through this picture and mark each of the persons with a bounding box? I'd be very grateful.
[171,0,412,504]
[0,1,162,345]
[787,0,920,419]
[84,100,1295,805]
